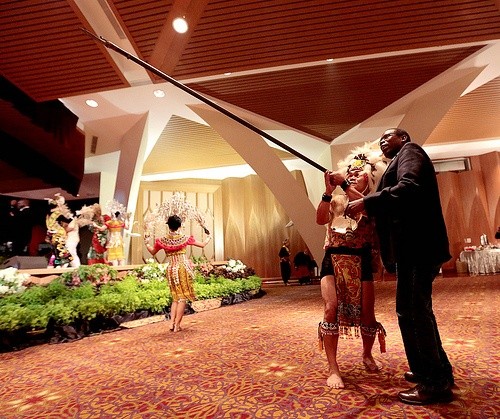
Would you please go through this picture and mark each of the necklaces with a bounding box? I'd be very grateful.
[361,185,368,194]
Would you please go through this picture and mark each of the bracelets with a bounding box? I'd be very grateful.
[127,222,130,225]
[205,235,213,243]
[322,192,333,203]
[146,244,150,247]
[340,178,351,192]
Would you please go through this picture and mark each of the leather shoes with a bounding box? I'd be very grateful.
[398,384,454,405]
[404,371,418,383]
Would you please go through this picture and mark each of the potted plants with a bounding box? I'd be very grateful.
[0,279,263,350]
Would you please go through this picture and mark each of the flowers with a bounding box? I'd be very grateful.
[140,257,167,281]
[191,255,219,277]
[226,260,247,274]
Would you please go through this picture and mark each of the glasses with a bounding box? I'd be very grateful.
[378,133,399,146]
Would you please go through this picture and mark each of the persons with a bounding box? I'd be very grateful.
[315,142,383,389]
[144,214,213,333]
[293,247,313,285]
[279,238,292,286]
[343,128,457,406]
[9,199,33,256]
[44,203,131,266]
